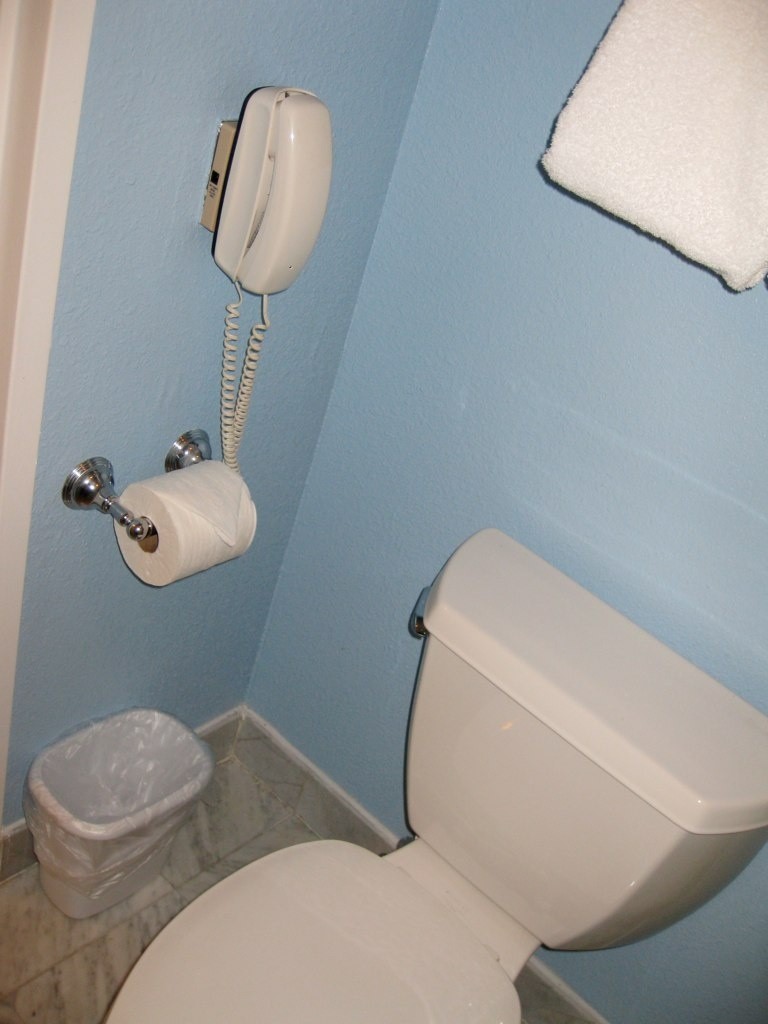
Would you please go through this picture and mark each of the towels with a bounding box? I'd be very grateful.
[535,1,767,292]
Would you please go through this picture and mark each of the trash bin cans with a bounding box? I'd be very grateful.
[22,707,215,915]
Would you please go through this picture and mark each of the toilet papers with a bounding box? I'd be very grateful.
[115,458,257,587]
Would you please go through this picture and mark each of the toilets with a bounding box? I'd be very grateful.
[96,528,766,1024]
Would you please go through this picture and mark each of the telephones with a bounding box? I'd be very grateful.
[210,86,335,297]
